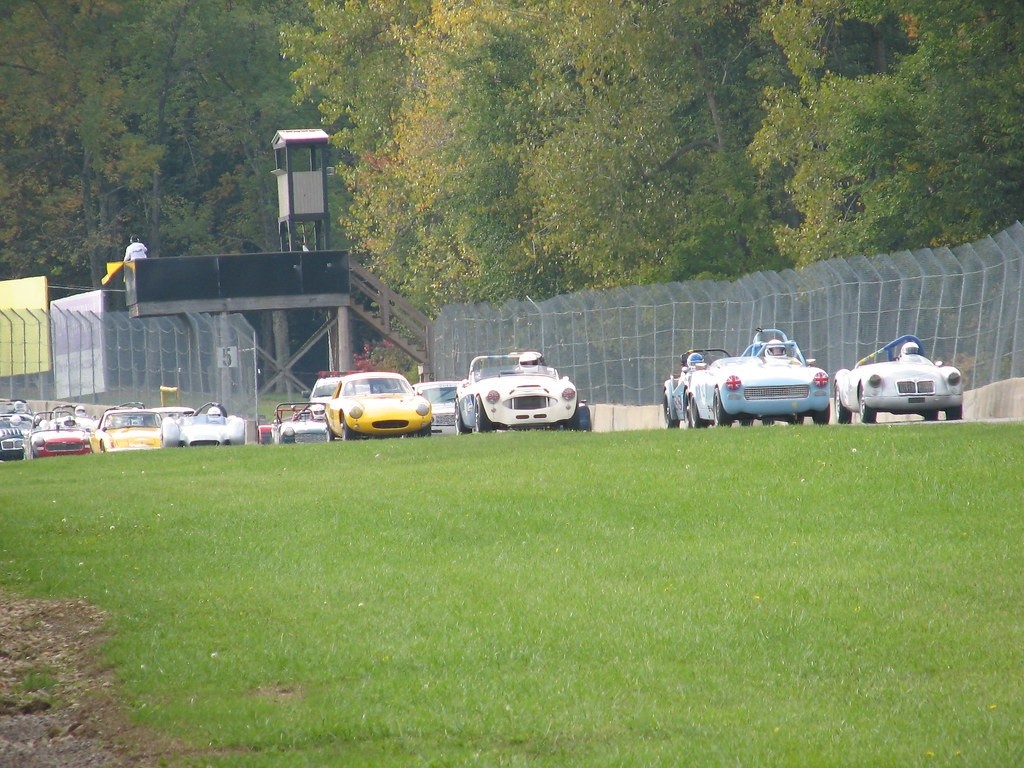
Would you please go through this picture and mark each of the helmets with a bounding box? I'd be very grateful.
[687,353,704,369]
[14,401,26,413]
[901,341,919,356]
[75,406,86,417]
[207,407,221,423]
[131,415,144,426]
[765,339,787,359]
[62,415,76,429]
[519,353,538,373]
[311,404,325,419]
[10,415,21,428]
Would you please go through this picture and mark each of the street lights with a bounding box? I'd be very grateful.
[526,295,544,356]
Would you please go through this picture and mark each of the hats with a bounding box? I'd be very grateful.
[130,235,139,243]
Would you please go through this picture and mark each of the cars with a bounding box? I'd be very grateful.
[412,381,460,436]
[663,329,831,428]
[0,398,245,461]
[456,351,592,436]
[272,370,434,444]
[833,335,964,424]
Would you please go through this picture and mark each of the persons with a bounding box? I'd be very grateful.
[686,352,704,366]
[74,406,88,417]
[207,406,221,423]
[896,342,919,361]
[764,339,787,359]
[59,415,76,430]
[518,353,538,373]
